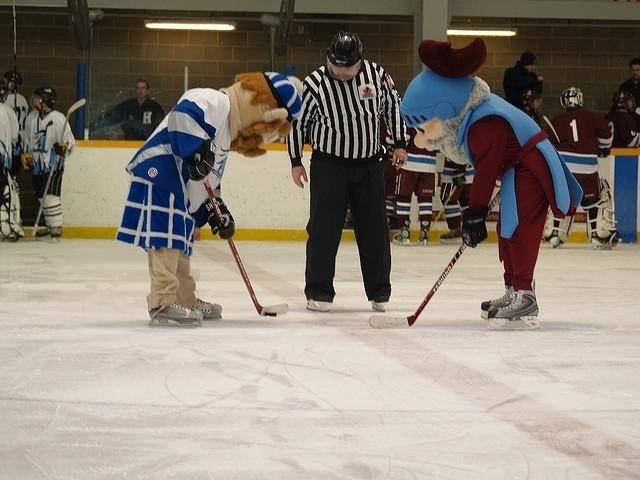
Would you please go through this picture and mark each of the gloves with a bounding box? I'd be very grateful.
[52,141,68,156]
[205,197,236,240]
[457,192,489,248]
[186,139,216,181]
[20,151,34,170]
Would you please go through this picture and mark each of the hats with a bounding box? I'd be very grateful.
[520,51,538,66]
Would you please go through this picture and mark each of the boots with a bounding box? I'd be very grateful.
[545,217,564,244]
[479,280,540,320]
[391,220,461,241]
[589,217,623,244]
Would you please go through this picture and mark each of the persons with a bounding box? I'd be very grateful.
[286,33,408,314]
[0,71,77,244]
[90,79,165,140]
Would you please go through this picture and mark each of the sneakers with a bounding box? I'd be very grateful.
[371,301,390,312]
[0,219,62,239]
[149,303,204,323]
[306,298,331,313]
[180,298,222,318]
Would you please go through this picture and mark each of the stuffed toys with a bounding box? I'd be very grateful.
[115,70,305,328]
[399,36,583,331]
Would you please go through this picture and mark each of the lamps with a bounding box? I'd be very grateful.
[444,17,520,38]
[141,9,237,33]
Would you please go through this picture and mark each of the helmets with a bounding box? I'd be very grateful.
[324,30,365,82]
[559,87,585,108]
[0,70,23,103]
[30,86,57,113]
[521,84,543,105]
[613,90,638,114]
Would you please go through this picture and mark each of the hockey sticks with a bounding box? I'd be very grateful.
[195,153,289,317]
[369,190,501,329]
[32,98,86,235]
